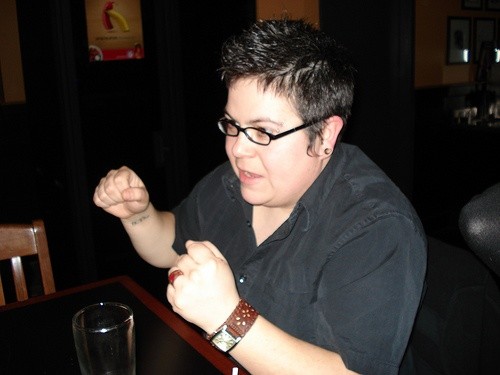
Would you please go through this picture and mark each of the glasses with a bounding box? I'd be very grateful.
[217,114,333,146]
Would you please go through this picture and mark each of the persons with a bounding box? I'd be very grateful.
[93,19,427,375]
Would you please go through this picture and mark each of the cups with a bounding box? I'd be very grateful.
[72,302,136,375]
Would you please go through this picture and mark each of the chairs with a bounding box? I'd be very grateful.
[399,237,500,375]
[0,219,56,306]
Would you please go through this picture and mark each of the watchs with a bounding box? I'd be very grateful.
[206,299,258,353]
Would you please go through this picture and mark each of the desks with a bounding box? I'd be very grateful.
[0,275,251,375]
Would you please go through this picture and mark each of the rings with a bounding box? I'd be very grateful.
[167,269,184,285]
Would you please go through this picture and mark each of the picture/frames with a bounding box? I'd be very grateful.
[447,0,500,66]
